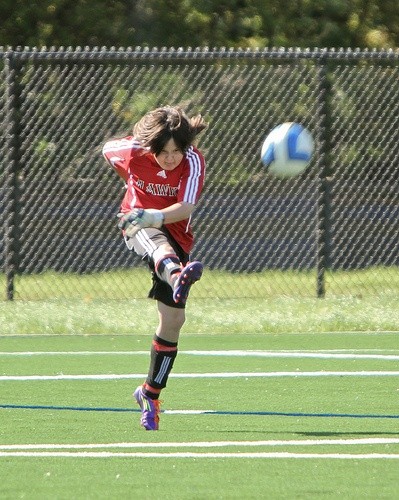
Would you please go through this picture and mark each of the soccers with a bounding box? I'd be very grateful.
[260,122,314,176]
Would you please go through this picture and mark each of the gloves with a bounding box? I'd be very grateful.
[116,208,165,241]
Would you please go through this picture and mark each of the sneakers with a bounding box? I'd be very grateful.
[173,261,203,305]
[132,385,160,430]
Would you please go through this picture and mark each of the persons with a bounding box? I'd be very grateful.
[100,104,208,431]
